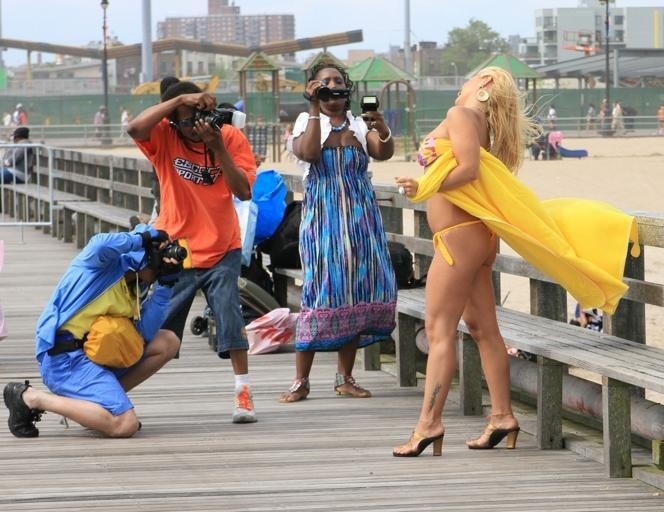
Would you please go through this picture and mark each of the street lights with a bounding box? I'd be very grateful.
[100,1,110,144]
[600,1,618,137]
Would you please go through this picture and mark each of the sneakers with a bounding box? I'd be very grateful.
[1,380,46,439]
[231,383,257,425]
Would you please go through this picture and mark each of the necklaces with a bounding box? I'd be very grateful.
[332,117,348,131]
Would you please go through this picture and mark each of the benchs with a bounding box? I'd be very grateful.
[2,144,90,237]
[59,154,154,250]
[277,171,664,479]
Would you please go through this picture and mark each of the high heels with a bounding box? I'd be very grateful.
[465,421,521,450]
[391,426,445,458]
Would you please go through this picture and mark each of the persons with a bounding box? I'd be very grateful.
[92,105,109,136]
[115,107,134,145]
[586,103,595,131]
[658,106,664,136]
[611,101,624,132]
[600,98,610,129]
[0,126,33,183]
[3,224,181,438]
[125,76,257,423]
[279,63,398,402]
[393,66,639,458]
[278,121,299,163]
[18,107,27,126]
[547,105,556,130]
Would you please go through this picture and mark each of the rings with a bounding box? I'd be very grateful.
[371,121,376,127]
[399,176,406,183]
[399,186,405,194]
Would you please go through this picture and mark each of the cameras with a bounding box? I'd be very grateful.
[315,84,349,102]
[360,95,379,110]
[156,242,187,265]
[193,107,247,131]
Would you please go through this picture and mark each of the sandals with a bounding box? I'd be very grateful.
[278,376,312,404]
[331,372,373,399]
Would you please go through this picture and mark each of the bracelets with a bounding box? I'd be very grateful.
[377,126,392,143]
[308,116,320,120]
